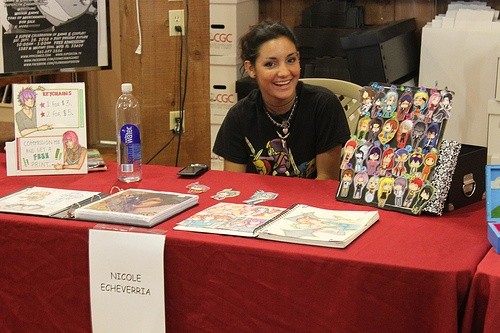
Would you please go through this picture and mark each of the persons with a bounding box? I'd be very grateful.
[213,19,351,181]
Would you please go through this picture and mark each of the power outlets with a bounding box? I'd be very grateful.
[169,9,184,36]
[170,110,185,133]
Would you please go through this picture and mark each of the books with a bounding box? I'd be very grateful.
[0,185,381,249]
[87,148,107,171]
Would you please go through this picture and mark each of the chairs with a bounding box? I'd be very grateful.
[297,78,364,139]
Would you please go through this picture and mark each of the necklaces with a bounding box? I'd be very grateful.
[263,95,299,133]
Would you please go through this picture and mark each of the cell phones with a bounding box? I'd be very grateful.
[176,163,208,177]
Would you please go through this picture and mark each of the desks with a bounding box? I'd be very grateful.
[0,149,500,333]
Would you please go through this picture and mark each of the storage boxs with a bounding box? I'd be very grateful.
[443,144,488,211]
[485,165,500,254]
[209,0,260,171]
[340,18,417,87]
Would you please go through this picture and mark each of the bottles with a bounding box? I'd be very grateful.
[115,83,141,182]
